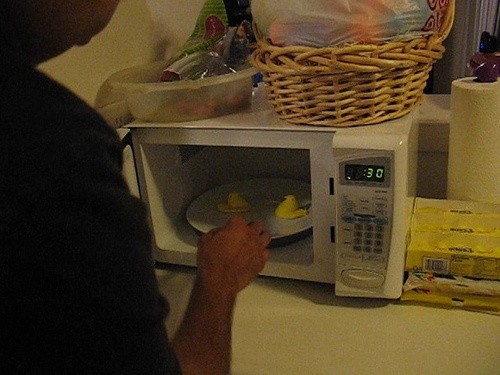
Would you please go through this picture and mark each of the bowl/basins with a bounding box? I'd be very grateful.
[108,60,260,122]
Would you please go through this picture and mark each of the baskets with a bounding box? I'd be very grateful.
[246,1,456,129]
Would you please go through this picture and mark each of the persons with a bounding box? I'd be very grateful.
[0,1,273,375]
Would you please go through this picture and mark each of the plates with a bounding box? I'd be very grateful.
[186,177,314,245]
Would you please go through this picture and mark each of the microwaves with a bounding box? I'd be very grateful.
[115,87,421,300]
